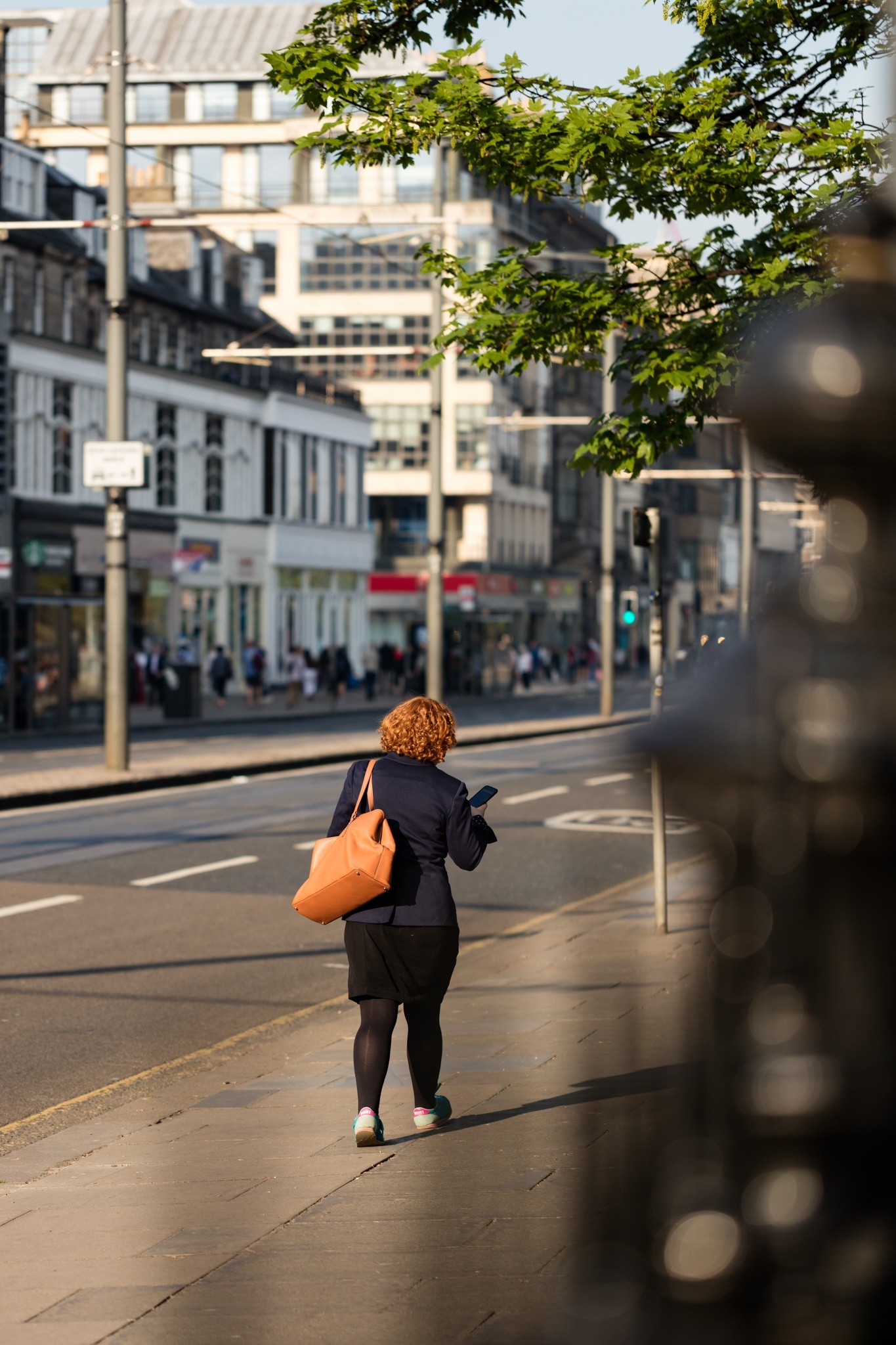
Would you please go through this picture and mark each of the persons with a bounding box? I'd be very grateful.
[145,637,649,711]
[327,697,499,1145]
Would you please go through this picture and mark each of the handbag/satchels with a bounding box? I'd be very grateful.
[292,760,395,925]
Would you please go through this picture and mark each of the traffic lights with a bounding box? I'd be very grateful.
[619,590,638,629]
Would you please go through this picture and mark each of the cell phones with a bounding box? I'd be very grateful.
[468,786,498,808]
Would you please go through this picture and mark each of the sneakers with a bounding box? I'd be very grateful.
[352,1114,386,1147]
[413,1083,453,1128]
